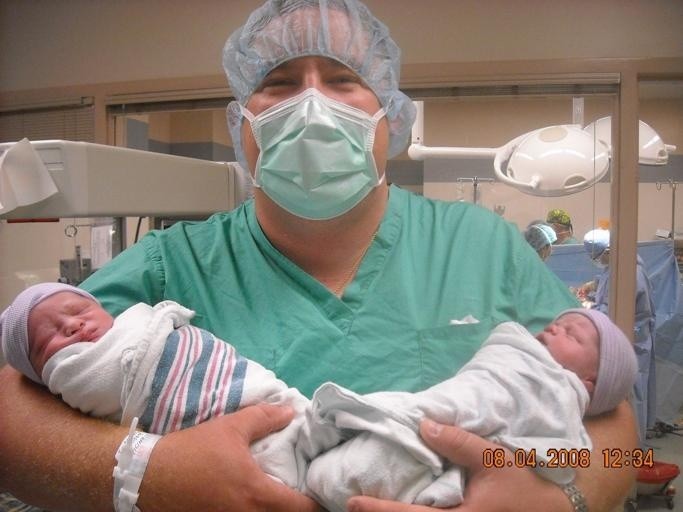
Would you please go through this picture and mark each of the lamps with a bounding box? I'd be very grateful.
[489,117,670,197]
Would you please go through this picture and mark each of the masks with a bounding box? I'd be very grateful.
[593,250,608,270]
[242,87,387,221]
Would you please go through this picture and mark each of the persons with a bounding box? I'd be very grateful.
[0,0,638,512]
[0,282,311,493]
[295,307,638,512]
[576,229,656,440]
[525,224,557,262]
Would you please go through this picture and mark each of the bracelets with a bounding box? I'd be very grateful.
[113,417,163,512]
[530,462,589,512]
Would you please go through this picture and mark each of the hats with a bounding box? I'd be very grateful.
[584,229,609,252]
[554,308,639,416]
[0,282,102,385]
